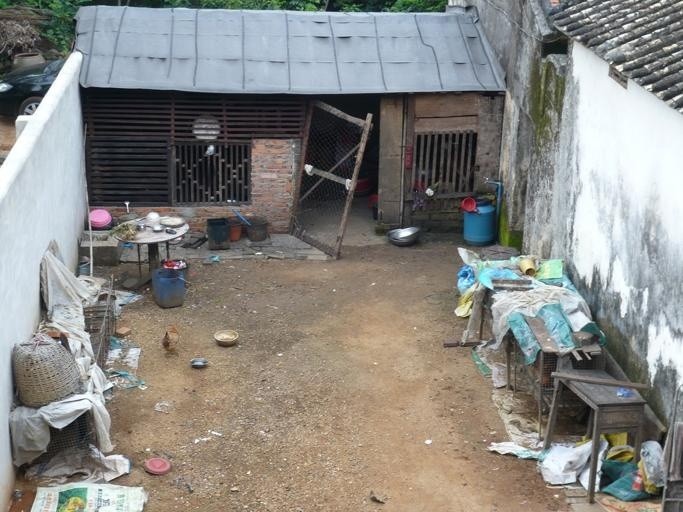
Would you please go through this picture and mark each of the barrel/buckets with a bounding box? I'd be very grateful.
[462,197,496,245]
[206,215,230,250]
[152,267,192,308]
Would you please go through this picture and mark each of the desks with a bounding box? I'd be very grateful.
[113,216,191,289]
[541,367,647,505]
[462,259,604,442]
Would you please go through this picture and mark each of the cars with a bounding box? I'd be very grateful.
[0,58,71,117]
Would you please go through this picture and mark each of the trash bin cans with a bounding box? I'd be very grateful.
[152,259,189,308]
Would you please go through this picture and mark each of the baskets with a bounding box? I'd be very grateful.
[14,335,83,408]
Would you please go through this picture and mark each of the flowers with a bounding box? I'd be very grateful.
[410,180,440,213]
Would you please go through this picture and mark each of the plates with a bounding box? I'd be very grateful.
[144,458,171,475]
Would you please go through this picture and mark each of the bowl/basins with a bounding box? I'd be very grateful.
[387,226,421,247]
[214,329,238,347]
[88,209,112,228]
[190,358,208,368]
